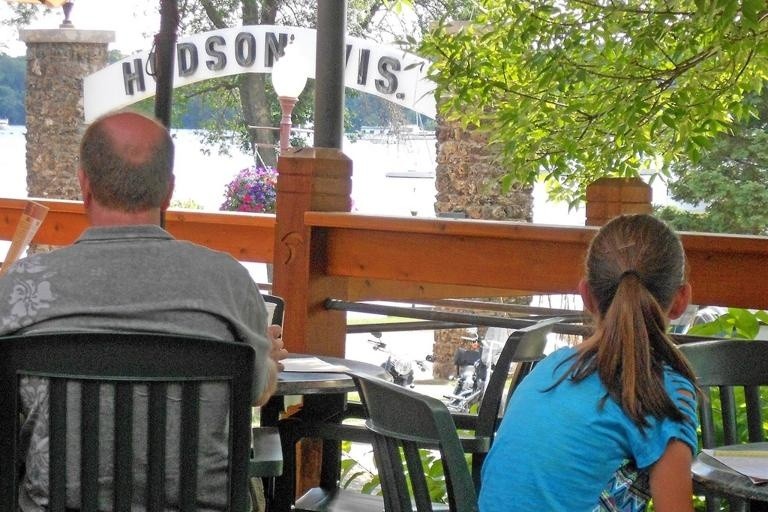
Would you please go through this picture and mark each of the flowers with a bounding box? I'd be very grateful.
[219,165,280,212]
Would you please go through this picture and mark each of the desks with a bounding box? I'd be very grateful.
[249,350,395,512]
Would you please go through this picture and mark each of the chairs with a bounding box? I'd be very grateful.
[2,330,257,512]
[352,373,480,512]
[675,336,768,511]
[260,295,287,338]
[273,317,566,512]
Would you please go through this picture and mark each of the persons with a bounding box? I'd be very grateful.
[477,214,708,511]
[1,110,288,511]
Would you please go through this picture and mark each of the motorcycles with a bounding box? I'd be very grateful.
[440,323,520,423]
[362,327,437,394]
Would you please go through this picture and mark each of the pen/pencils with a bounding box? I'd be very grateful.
[713,450,768,457]
